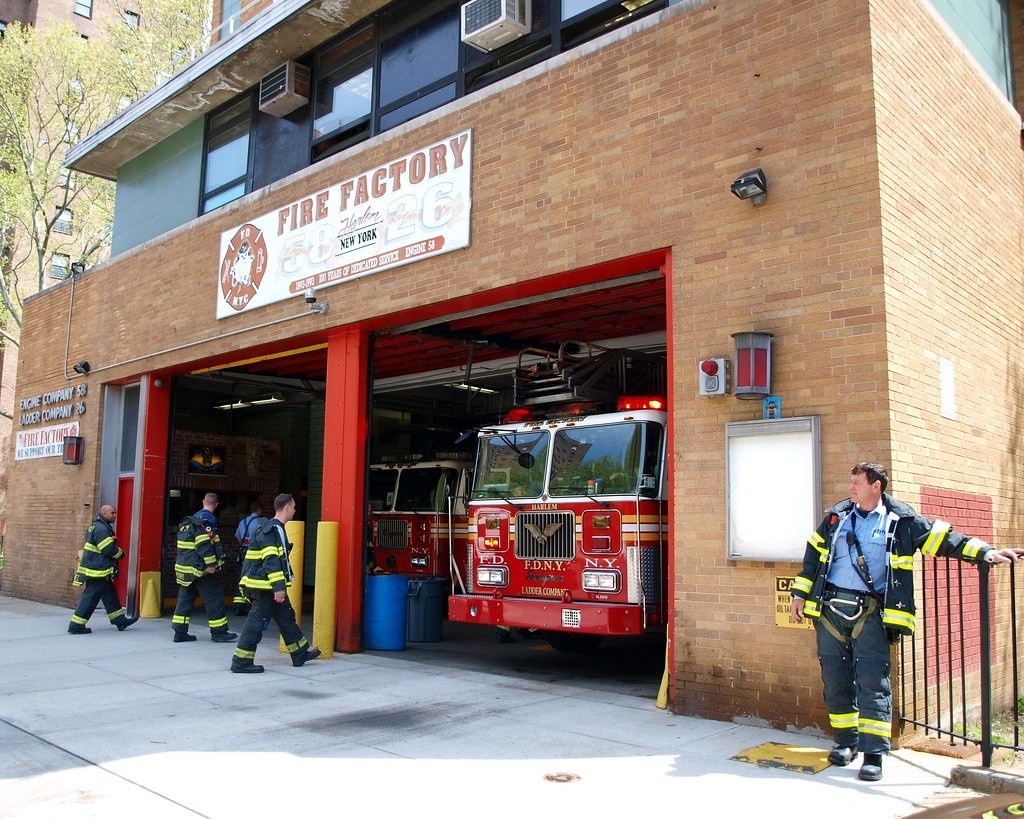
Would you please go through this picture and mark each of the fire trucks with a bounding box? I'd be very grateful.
[448,339,667,679]
[364,451,512,619]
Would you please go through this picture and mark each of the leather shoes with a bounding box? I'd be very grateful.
[858,754,882,781]
[173,630,197,643]
[828,744,858,766]
[117,617,139,631]
[230,661,264,673]
[68,627,92,634]
[212,632,237,641]
[292,648,321,667]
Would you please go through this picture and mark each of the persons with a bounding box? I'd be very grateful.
[170,493,239,642]
[229,493,321,674]
[66,505,140,634]
[367,500,391,576]
[233,501,270,617]
[788,461,1024,780]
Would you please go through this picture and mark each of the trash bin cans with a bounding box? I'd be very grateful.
[364,574,409,653]
[407,578,452,643]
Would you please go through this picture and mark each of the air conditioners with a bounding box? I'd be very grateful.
[460,0,532,53]
[259,60,311,118]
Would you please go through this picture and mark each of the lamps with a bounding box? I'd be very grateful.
[731,168,767,205]
[730,331,776,400]
[304,287,328,313]
[71,262,85,274]
[73,361,89,376]
[62,436,84,465]
[212,392,286,410]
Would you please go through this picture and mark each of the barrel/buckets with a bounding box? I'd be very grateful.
[363,573,409,651]
